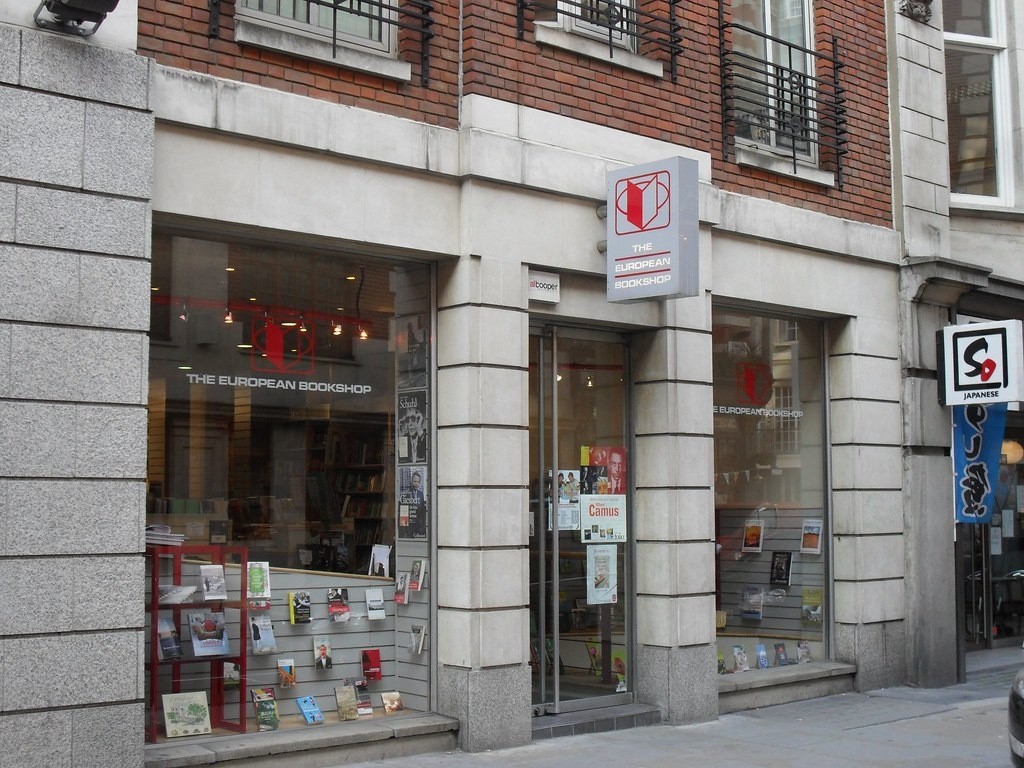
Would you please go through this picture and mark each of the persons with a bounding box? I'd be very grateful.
[398,401,425,463]
[251,616,263,652]
[205,577,212,593]
[550,472,580,505]
[400,470,427,538]
[316,644,332,670]
[396,576,406,595]
[408,322,421,369]
[582,446,627,495]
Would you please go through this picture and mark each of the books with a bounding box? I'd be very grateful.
[144,410,429,739]
[530,519,824,675]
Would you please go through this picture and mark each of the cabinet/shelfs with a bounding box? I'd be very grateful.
[309,420,395,579]
[146,544,247,744]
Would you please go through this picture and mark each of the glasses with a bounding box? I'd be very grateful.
[405,415,418,423]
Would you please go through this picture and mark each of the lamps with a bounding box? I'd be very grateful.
[224,301,234,324]
[358,325,368,340]
[299,312,307,333]
